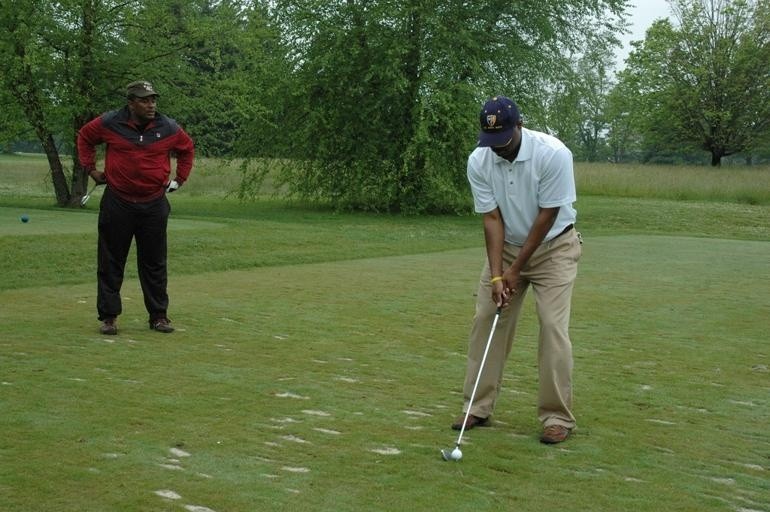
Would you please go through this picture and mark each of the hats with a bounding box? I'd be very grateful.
[476,96,519,147]
[127,81,160,98]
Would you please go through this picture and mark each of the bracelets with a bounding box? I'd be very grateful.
[489,276,504,285]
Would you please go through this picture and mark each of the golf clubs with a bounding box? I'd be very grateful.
[441,296,504,464]
[79,173,105,208]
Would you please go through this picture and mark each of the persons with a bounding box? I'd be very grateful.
[76,79,196,335]
[448,96,583,446]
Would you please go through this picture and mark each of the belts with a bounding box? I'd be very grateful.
[553,224,573,239]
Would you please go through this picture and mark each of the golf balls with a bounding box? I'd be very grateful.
[21,215,29,222]
[451,448,463,460]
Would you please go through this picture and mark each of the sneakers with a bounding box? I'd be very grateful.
[100,319,117,335]
[149,318,173,333]
[539,424,571,442]
[452,413,491,430]
